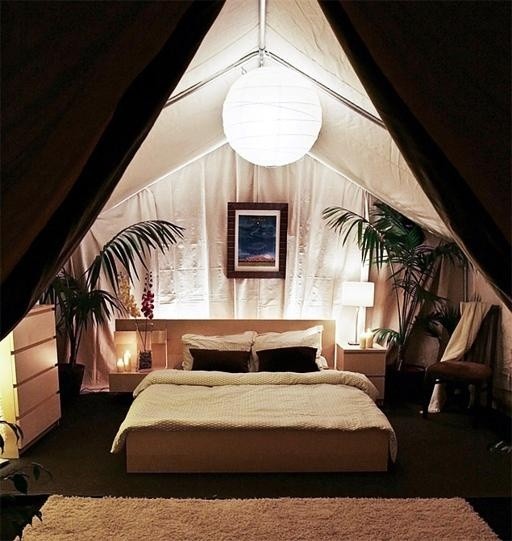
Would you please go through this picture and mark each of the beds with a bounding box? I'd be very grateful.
[110,319,390,473]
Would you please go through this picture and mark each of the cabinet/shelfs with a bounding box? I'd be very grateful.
[0,304,64,461]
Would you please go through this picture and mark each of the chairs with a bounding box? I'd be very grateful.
[426,300,500,428]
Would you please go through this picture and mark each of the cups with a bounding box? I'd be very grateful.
[360,333,373,349]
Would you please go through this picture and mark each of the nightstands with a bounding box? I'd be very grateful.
[336,341,387,406]
[108,330,169,393]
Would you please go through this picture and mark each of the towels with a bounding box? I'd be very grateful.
[427,301,493,414]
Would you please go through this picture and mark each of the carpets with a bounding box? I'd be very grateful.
[15,494,502,541]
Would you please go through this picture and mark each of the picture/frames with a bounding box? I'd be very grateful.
[226,201,289,279]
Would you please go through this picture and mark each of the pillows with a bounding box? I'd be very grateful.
[173,323,329,372]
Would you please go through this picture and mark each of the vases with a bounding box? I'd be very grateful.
[136,350,153,372]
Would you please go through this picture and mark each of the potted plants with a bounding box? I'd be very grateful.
[321,203,469,416]
[39,220,187,396]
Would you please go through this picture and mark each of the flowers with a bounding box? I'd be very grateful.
[115,270,155,352]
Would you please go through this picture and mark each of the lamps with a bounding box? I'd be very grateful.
[339,281,375,346]
[221,52,323,167]
